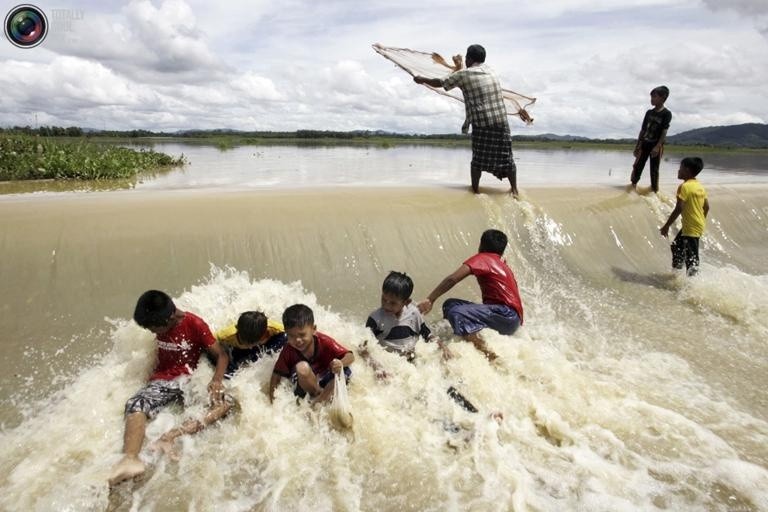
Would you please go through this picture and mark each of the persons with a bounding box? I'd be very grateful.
[414,43,519,202]
[630,86,672,193]
[662,155,710,274]
[268,305,355,415]
[200,311,288,380]
[359,271,480,420]
[107,288,236,484]
[417,230,523,364]
